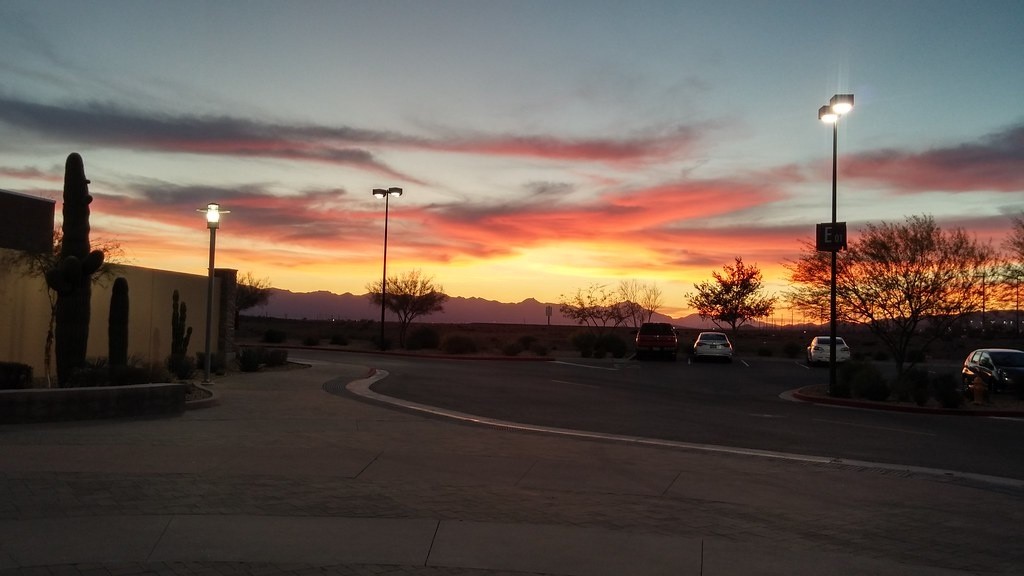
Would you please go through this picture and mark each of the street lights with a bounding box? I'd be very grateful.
[373,187,402,351]
[818,92,855,396]
[196,202,232,387]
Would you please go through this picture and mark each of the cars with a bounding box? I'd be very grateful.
[805,336,851,367]
[961,348,1024,402]
[692,332,733,363]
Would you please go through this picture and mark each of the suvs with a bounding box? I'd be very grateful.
[630,322,680,362]
